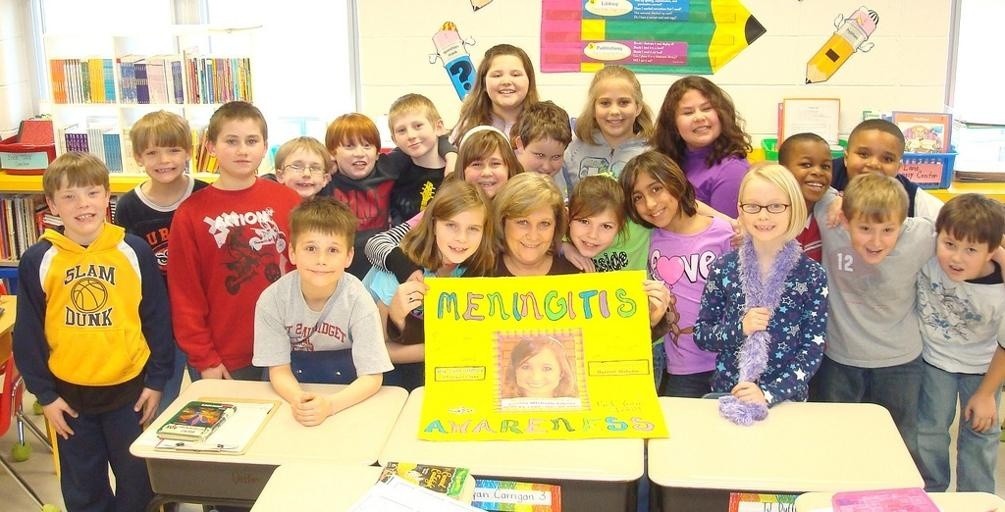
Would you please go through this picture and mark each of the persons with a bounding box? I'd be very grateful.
[15,44,1005,510]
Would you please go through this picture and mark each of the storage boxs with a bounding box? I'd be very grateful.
[761,136,849,161]
[865,150,963,190]
[0,134,57,176]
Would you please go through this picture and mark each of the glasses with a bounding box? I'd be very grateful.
[738,199,795,215]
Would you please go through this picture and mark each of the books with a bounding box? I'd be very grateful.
[1,58,251,295]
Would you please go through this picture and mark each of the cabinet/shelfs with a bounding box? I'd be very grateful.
[0,170,220,268]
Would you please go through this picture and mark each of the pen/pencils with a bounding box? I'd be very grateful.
[431,22,477,100]
[803,8,881,85]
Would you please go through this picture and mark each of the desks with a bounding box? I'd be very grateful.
[128,377,1005,512]
[0,294,17,368]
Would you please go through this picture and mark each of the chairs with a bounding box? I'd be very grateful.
[1,349,61,512]
